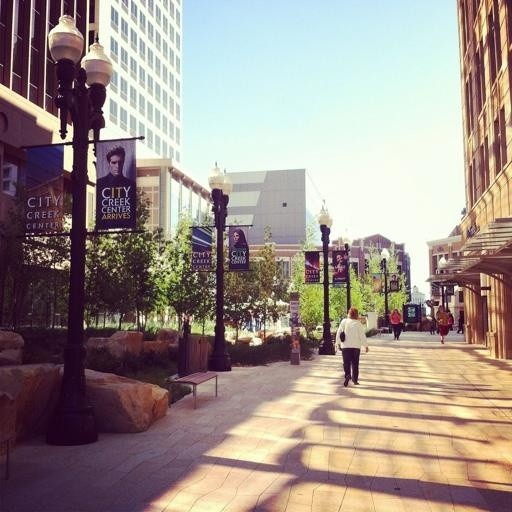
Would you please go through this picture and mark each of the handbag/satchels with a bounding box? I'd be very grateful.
[340,319,346,342]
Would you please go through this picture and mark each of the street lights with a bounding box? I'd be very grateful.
[438,257,446,311]
[48,15,114,447]
[379,248,390,331]
[317,199,337,356]
[341,226,353,314]
[207,160,233,372]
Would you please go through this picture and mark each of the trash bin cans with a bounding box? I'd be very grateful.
[188,336,209,373]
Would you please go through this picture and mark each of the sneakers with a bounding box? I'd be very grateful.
[343,375,350,387]
[354,382,359,385]
[441,341,444,344]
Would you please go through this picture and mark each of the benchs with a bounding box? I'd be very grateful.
[168,372,220,410]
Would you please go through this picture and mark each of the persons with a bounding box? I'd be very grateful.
[336,308,369,387]
[182,320,191,336]
[234,230,249,265]
[457,316,464,334]
[390,275,398,290]
[333,255,346,280]
[431,305,454,344]
[391,309,401,340]
[97,146,134,223]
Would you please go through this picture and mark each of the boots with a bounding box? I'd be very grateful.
[394,334,399,340]
